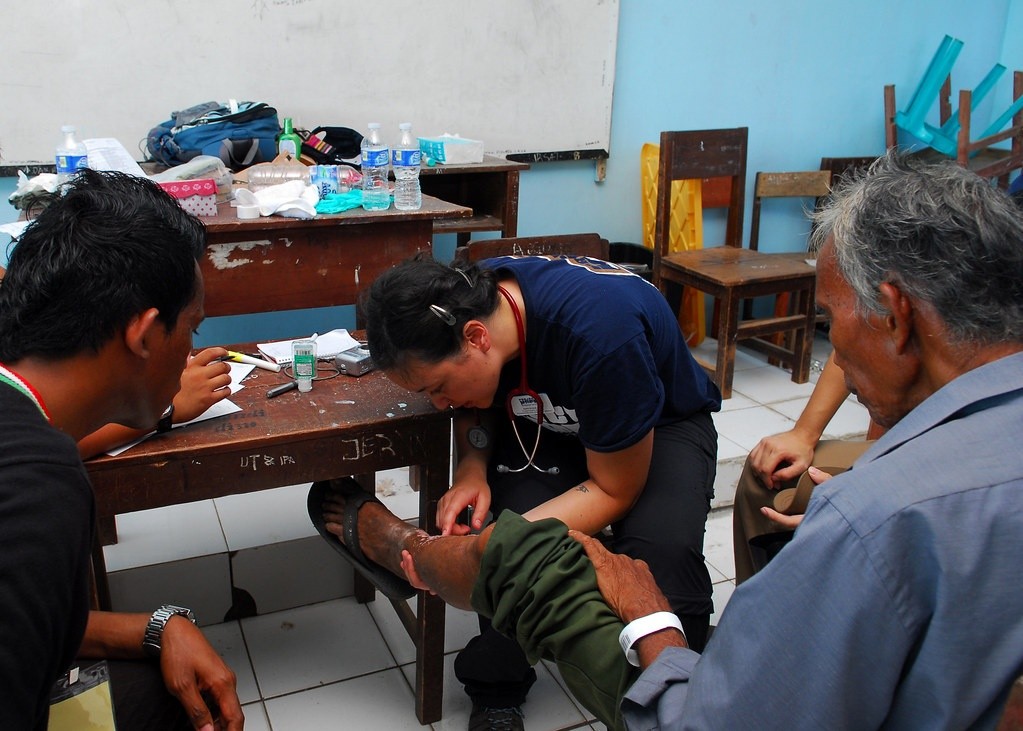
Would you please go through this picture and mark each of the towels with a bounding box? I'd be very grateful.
[234,178,320,218]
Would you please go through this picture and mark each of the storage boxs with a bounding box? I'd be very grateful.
[158,178,220,217]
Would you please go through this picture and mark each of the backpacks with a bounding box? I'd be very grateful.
[138,99,282,170]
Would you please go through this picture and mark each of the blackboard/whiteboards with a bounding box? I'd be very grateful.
[0,0,620,176]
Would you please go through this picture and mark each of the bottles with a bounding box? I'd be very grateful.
[278,118,301,162]
[246,163,372,196]
[360,121,391,212]
[55,126,88,197]
[391,122,422,210]
[291,339,318,394]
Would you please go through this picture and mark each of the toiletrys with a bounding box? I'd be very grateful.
[278,117,301,161]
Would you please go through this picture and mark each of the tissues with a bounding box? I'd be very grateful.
[417,134,484,164]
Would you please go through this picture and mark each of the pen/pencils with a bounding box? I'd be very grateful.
[212,356,237,362]
[266,380,297,399]
[226,350,282,372]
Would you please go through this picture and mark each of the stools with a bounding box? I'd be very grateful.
[895,35,1023,158]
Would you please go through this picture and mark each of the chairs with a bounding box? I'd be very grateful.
[653,70,1023,400]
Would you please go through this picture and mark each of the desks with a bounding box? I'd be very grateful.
[9,154,530,728]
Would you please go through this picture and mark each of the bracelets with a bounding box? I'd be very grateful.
[618,610,689,667]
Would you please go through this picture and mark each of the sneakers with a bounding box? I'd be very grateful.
[467,704,526,731]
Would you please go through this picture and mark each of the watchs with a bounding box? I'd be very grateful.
[141,604,197,681]
[157,403,174,432]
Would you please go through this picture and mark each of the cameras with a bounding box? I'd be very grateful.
[335,342,375,377]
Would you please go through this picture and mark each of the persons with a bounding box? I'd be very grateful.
[307,151,1023,731]
[360,256,722,731]
[0,163,246,731]
[735,175,1023,587]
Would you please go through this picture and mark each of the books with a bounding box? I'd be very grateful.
[256,330,359,367]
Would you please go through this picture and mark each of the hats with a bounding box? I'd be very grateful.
[773,466,850,517]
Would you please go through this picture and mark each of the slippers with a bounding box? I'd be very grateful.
[307,476,419,600]
